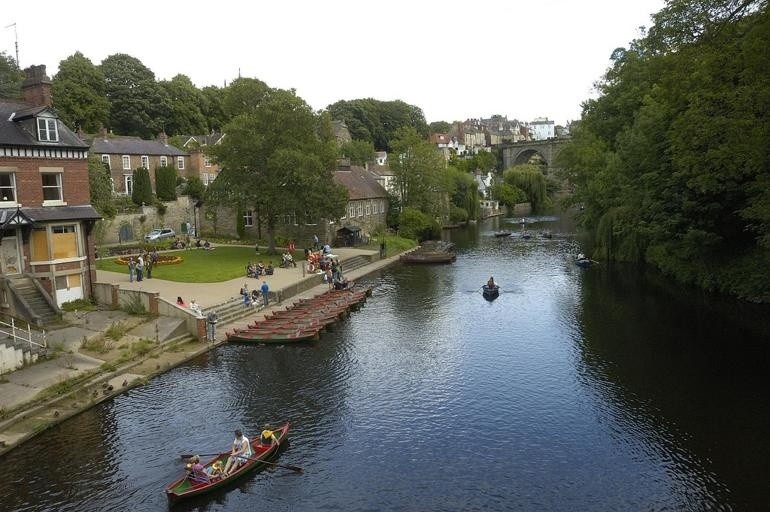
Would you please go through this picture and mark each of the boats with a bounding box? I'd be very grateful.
[225,286,373,345]
[483,285,499,296]
[574,258,589,266]
[165,424,290,505]
[400,240,456,263]
[494,218,551,239]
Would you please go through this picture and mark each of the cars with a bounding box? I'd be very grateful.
[144,228,175,242]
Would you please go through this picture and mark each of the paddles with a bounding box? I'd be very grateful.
[234,456,304,473]
[181,453,232,457]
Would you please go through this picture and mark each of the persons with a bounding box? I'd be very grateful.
[488,278,494,289]
[186,457,195,472]
[206,311,219,341]
[125,220,212,282]
[241,231,350,308]
[260,423,280,446]
[191,455,220,482]
[577,251,585,260]
[188,297,201,314]
[176,296,187,309]
[219,429,253,476]
